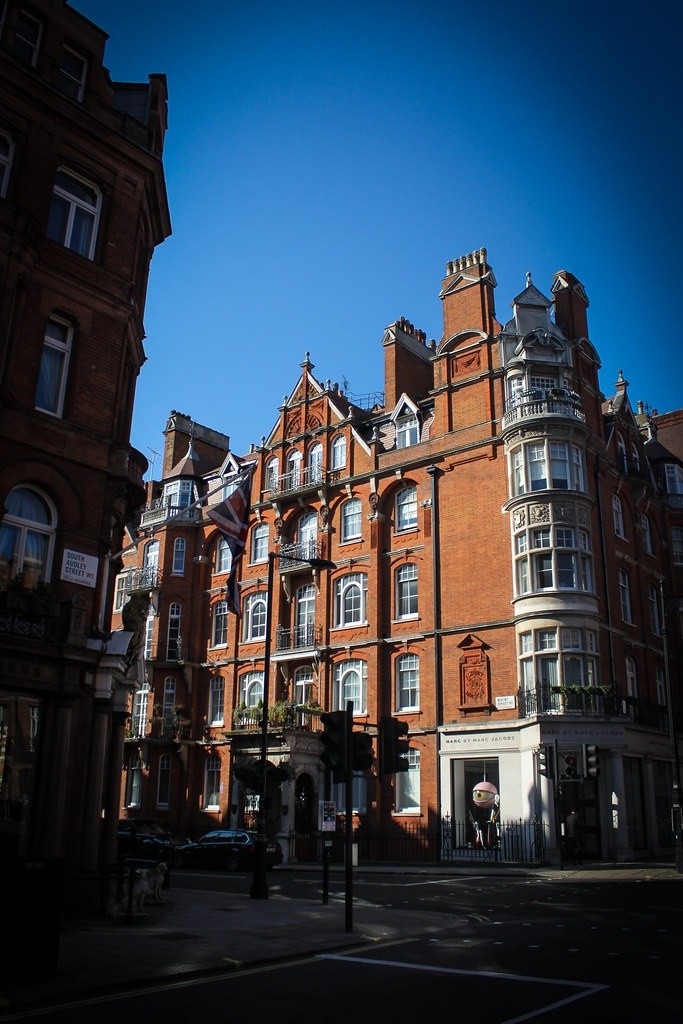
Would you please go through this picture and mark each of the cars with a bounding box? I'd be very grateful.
[117,818,190,862]
[179,829,284,876]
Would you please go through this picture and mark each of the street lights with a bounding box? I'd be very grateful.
[249,552,340,893]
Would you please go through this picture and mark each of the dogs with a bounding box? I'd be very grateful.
[113,861,170,912]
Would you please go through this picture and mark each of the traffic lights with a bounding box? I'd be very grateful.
[537,745,552,779]
[565,754,577,778]
[582,742,601,779]
[352,730,373,772]
[380,715,410,777]
[318,709,347,772]
